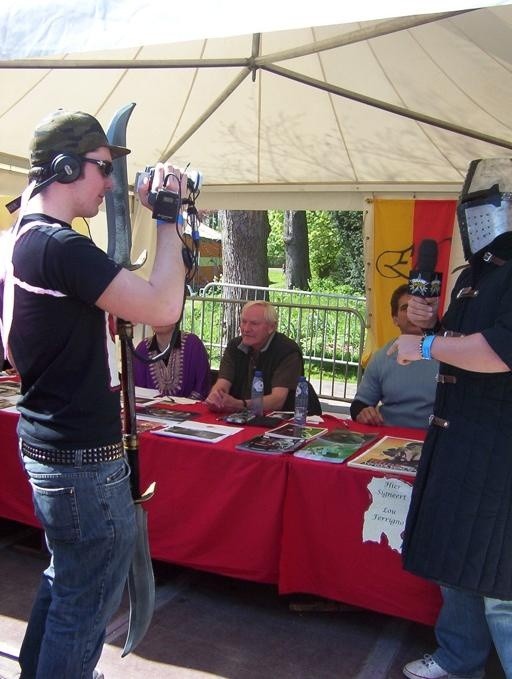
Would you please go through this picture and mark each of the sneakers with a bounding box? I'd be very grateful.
[402,654,484,679]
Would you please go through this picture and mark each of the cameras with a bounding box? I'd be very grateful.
[134,164,204,211]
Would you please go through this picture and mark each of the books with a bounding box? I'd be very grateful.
[121,385,425,479]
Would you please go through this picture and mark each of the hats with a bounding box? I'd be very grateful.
[28,111,131,168]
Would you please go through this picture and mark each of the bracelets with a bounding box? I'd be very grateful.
[239,397,247,413]
[156,213,185,225]
[419,335,427,360]
[423,334,436,360]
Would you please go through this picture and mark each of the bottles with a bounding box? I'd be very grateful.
[251,370,263,417]
[293,377,310,426]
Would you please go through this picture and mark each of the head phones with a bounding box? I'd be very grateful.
[3,149,82,215]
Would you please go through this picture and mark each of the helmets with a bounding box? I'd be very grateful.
[457,157,512,261]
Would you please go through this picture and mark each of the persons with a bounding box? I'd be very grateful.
[133,322,210,401]
[349,281,439,431]
[205,299,304,414]
[0,106,191,679]
[385,158,512,679]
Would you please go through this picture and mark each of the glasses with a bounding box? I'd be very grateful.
[80,155,113,177]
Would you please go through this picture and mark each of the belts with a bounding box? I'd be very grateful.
[21,440,125,464]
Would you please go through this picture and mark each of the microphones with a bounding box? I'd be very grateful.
[414,238,437,300]
